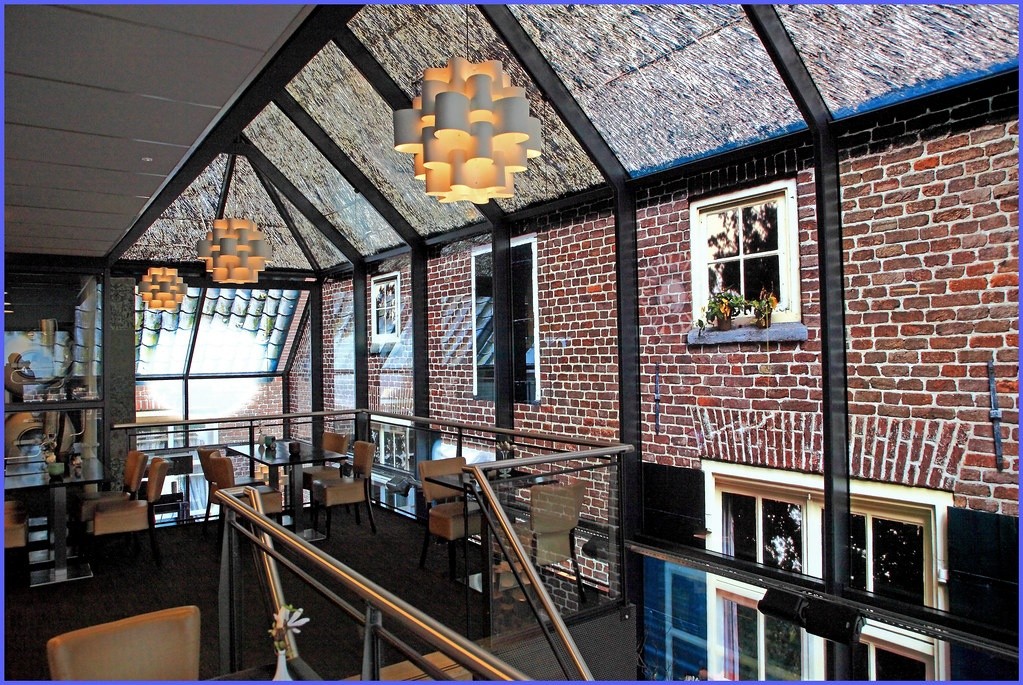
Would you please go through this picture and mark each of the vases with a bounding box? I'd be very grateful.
[272,650,293,680]
[758,317,772,328]
[499,451,512,477]
[258,434,267,444]
[716,317,731,331]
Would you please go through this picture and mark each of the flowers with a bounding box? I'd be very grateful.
[255,419,265,435]
[754,290,778,328]
[496,441,517,451]
[264,603,310,653]
[693,287,749,337]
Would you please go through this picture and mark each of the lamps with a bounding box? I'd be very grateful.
[393,4,543,204]
[756,587,805,626]
[194,155,273,284]
[139,259,188,310]
[581,538,608,559]
[385,477,411,498]
[805,598,866,648]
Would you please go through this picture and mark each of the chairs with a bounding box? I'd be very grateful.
[311,440,378,540]
[87,456,170,577]
[197,445,265,532]
[493,481,587,616]
[68,449,148,553]
[47,606,201,682]
[209,450,284,550]
[418,457,488,583]
[289,432,351,520]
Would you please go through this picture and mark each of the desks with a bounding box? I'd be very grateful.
[425,470,559,594]
[4,443,116,586]
[227,439,348,542]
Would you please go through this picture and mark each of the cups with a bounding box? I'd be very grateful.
[289,442,301,456]
[48,462,64,478]
[57,452,69,467]
[265,436,276,448]
